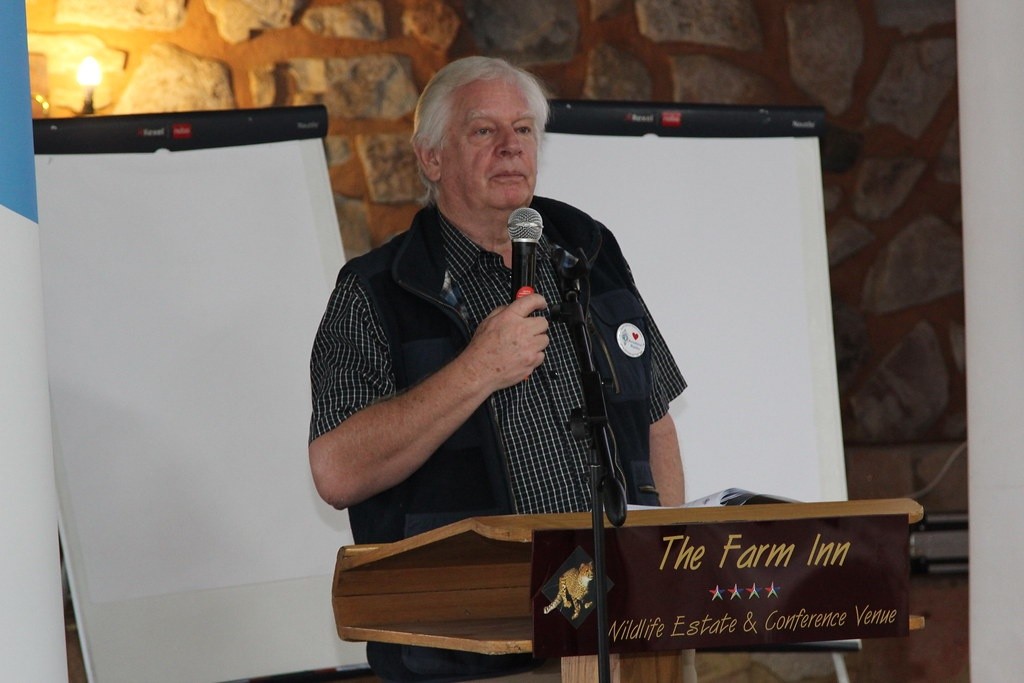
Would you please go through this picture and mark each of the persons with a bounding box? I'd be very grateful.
[309,57,689,683]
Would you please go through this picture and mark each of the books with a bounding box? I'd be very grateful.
[602,487,800,511]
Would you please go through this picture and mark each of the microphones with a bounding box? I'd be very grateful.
[508,207,543,306]
